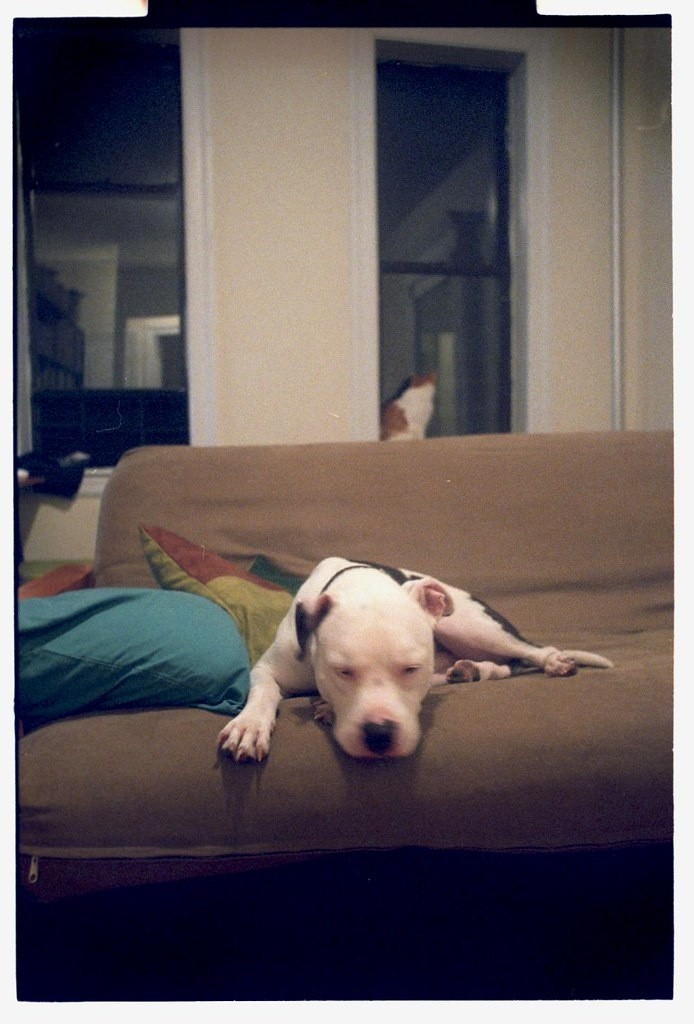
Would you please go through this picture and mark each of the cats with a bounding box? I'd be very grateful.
[382,375,440,441]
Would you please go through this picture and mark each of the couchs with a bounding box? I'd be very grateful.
[15,427,672,905]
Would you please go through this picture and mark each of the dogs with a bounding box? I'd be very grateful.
[217,553,616,763]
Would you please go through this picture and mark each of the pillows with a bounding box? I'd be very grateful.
[136,521,309,661]
[17,588,249,721]
